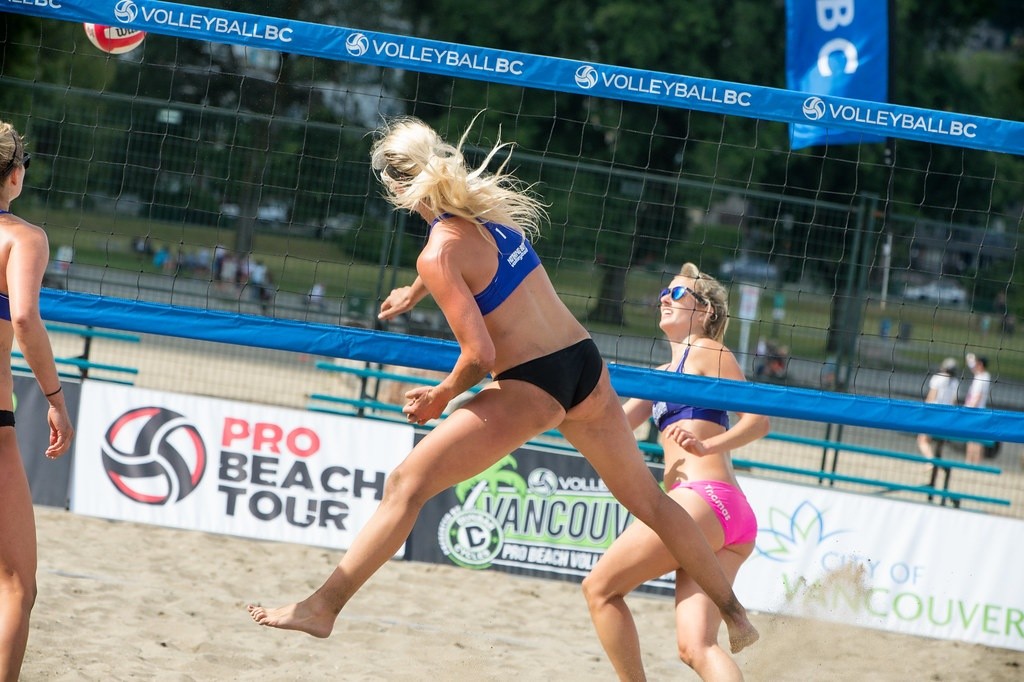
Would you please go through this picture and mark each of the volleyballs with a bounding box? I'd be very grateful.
[83,20,148,55]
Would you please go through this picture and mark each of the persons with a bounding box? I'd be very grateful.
[0,122,76,682]
[765,293,1012,473]
[246,114,761,656]
[581,264,769,682]
[56,234,330,317]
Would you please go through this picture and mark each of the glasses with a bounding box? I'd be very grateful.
[658,286,715,317]
[20,151,32,169]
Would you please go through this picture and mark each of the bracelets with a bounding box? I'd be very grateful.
[45,387,61,397]
